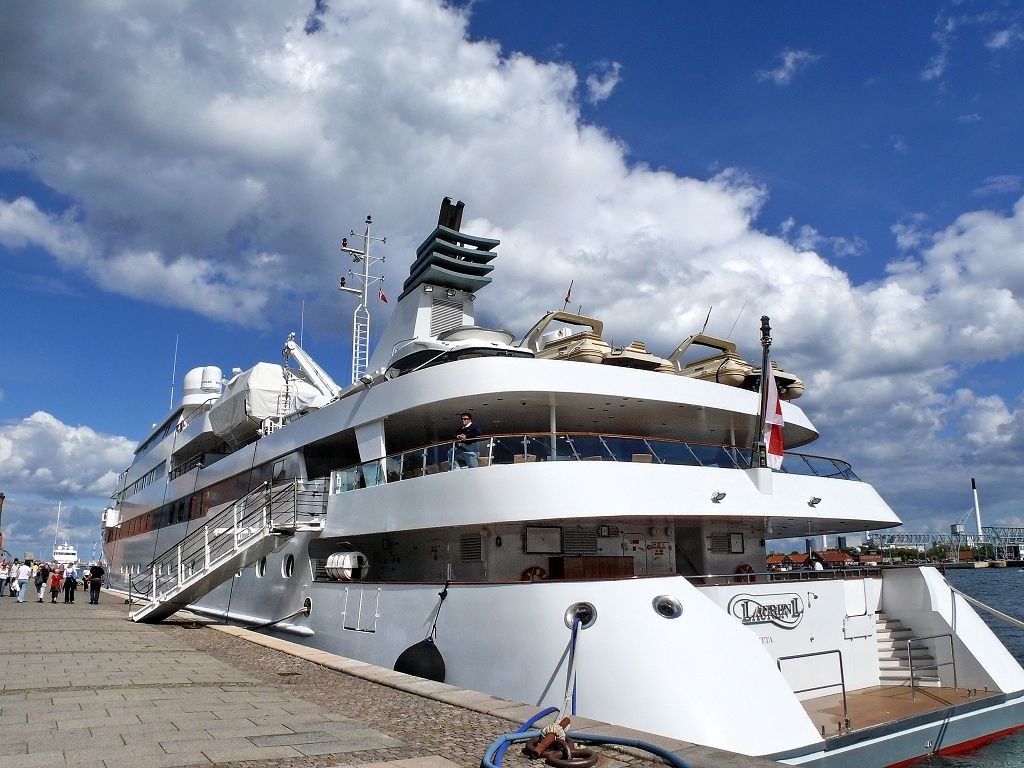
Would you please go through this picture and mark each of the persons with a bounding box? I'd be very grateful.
[448,412,481,472]
[0,557,106,605]
[812,558,823,570]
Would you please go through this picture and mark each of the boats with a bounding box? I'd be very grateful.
[83,541,101,581]
[99,196,1024,768]
[46,499,83,578]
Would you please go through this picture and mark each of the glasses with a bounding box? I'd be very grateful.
[2,565,6,566]
[461,418,469,420]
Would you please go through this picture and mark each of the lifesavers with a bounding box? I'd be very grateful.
[521,566,550,581]
[735,564,756,582]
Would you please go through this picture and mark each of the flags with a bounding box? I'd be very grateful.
[378,290,388,304]
[762,361,786,471]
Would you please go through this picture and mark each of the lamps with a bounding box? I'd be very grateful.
[496,537,501,546]
[469,295,476,301]
[424,285,433,292]
[809,496,821,504]
[446,289,456,296]
[713,492,726,500]
[761,539,765,546]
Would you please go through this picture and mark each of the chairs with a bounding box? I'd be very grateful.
[393,454,602,480]
[632,454,653,463]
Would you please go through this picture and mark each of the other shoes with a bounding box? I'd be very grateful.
[54,601,56,604]
[94,601,98,604]
[70,602,74,604]
[40,599,42,603]
[90,602,93,604]
[66,602,69,604]
[52,600,54,603]
[18,598,23,603]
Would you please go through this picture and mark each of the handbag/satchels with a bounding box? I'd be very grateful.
[35,574,43,586]
